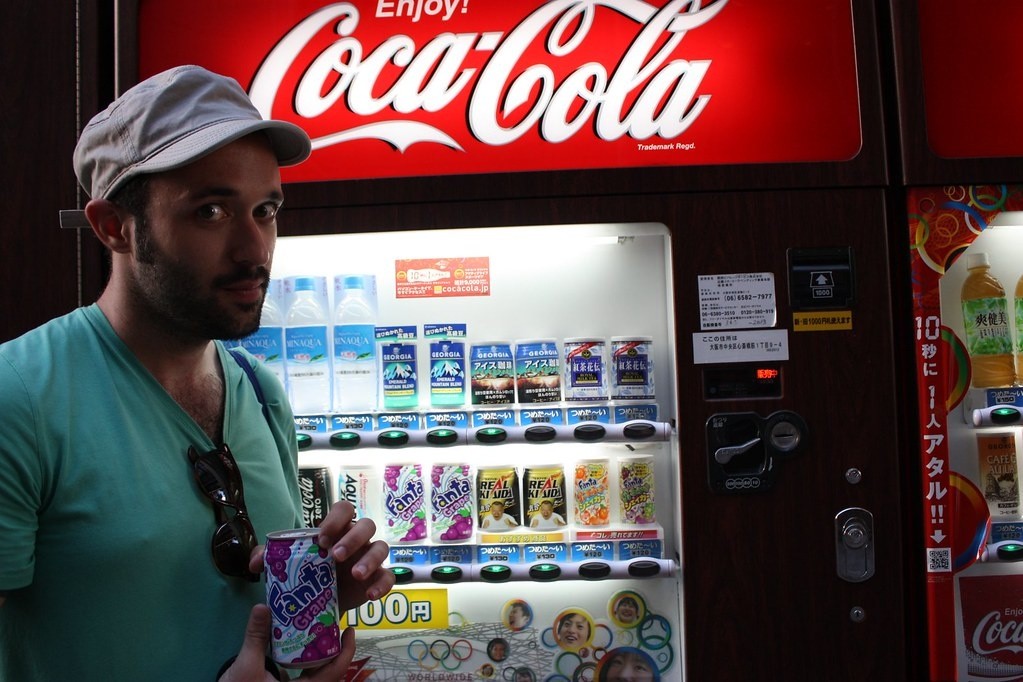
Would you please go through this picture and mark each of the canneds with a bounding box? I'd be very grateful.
[264,528,342,668]
[296,464,567,544]
[382,335,656,412]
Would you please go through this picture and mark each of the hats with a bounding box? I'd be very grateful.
[73,64,311,201]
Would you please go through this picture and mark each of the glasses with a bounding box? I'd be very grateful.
[187,442,261,582]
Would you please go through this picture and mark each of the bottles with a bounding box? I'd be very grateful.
[962,253,1017,388]
[1014,275,1023,387]
[240,280,286,394]
[334,277,379,413]
[285,277,330,415]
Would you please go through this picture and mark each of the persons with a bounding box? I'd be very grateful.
[0,64,394,682]
[558,613,590,646]
[482,666,493,676]
[614,598,639,623]
[530,501,566,528]
[600,652,654,682]
[509,604,530,627]
[481,501,518,530]
[515,671,532,682]
[492,642,506,660]
